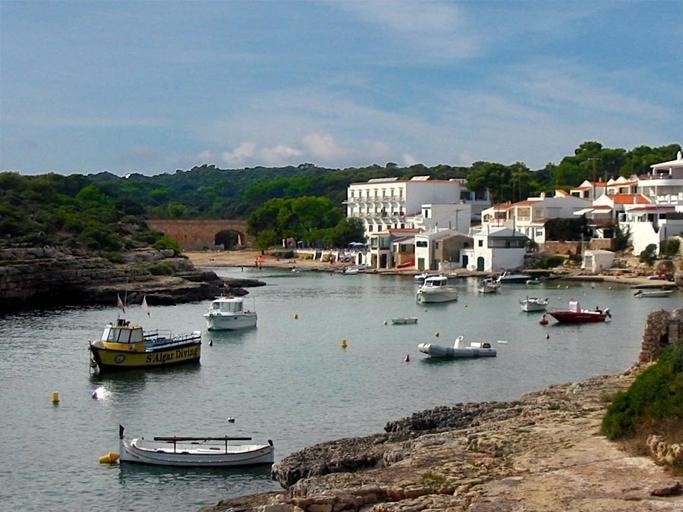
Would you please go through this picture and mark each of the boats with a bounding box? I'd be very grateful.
[115,424,278,470]
[633,288,675,301]
[391,317,418,326]
[336,267,360,275]
[88,316,203,369]
[202,292,260,332]
[414,270,613,326]
[416,342,498,360]
